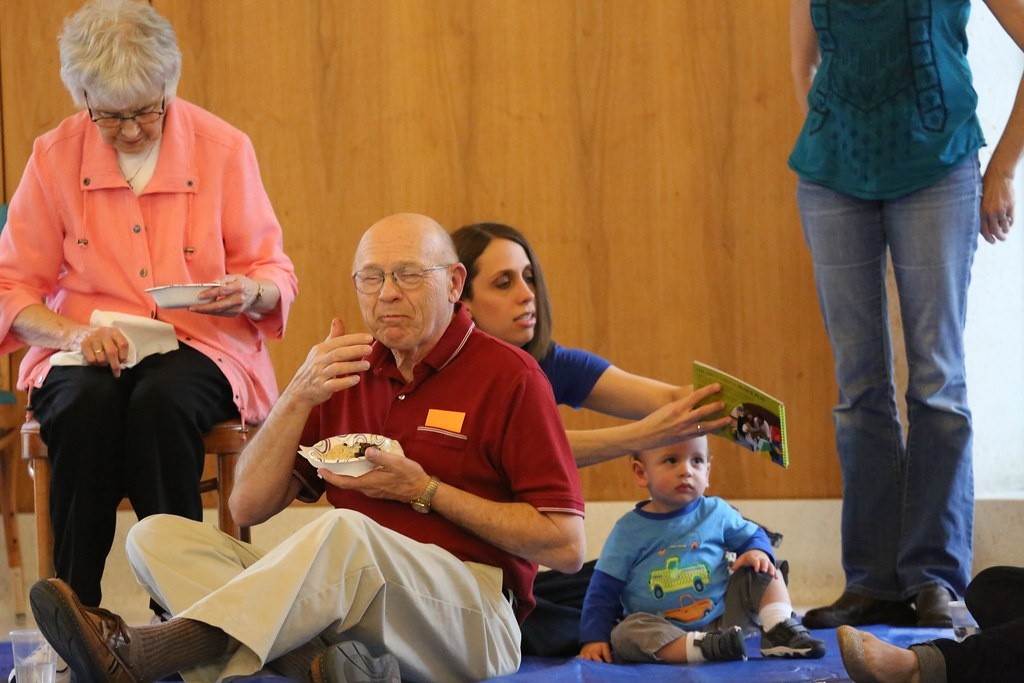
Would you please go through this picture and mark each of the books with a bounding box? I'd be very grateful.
[692,360,789,468]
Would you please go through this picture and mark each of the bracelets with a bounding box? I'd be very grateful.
[251,283,264,305]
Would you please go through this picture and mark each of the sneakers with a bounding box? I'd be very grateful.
[30,577,146,683]
[693,624,747,662]
[759,610,826,658]
[304,640,403,683]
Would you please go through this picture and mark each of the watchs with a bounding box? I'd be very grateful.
[409,475,440,513]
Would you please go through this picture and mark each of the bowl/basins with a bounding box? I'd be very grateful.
[309,433,387,474]
[144,283,226,308]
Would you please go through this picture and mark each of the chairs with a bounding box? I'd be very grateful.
[20,399,252,611]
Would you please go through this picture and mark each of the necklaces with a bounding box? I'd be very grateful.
[127,141,156,182]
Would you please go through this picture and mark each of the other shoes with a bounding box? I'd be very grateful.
[802,589,918,630]
[911,584,953,628]
[837,625,885,683]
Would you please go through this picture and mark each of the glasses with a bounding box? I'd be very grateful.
[83,85,166,128]
[352,263,453,296]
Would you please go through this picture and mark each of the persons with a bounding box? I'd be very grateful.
[835,566,1024,683]
[737,412,773,452]
[785,0,1024,629]
[0,0,298,683]
[576,433,827,665]
[447,222,733,659]
[30,214,587,683]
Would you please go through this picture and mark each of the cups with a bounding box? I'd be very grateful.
[10,630,57,683]
[949,601,975,643]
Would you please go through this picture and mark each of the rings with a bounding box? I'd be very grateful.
[94,349,103,354]
[698,424,701,434]
